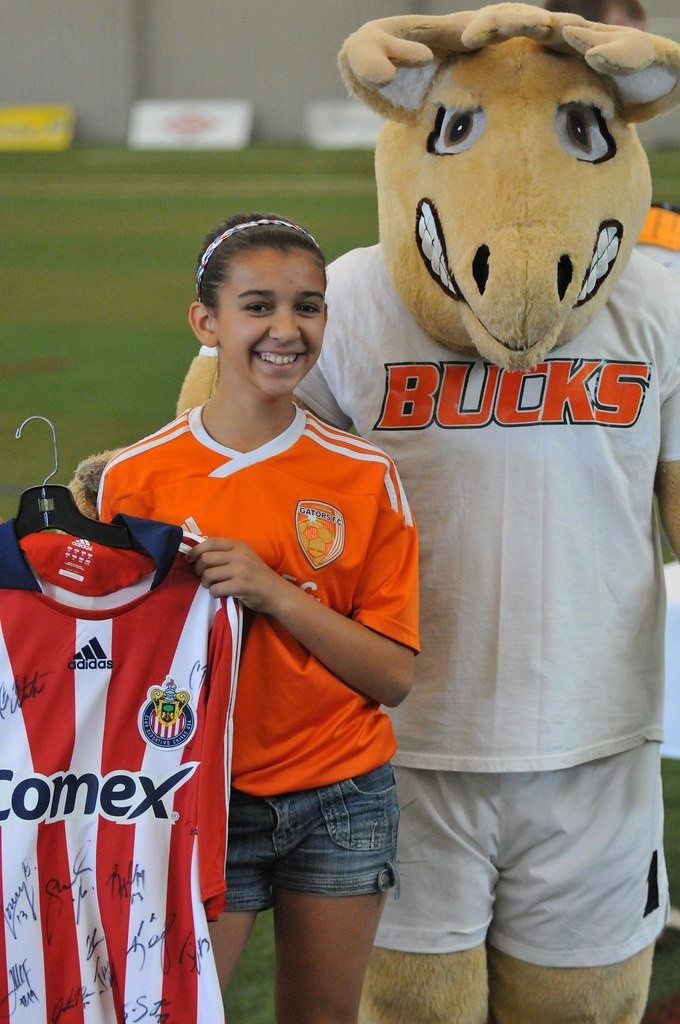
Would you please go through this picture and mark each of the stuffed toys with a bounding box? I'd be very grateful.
[67,6,680,1024]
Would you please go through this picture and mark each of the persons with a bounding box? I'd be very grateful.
[98,215,421,1024]
